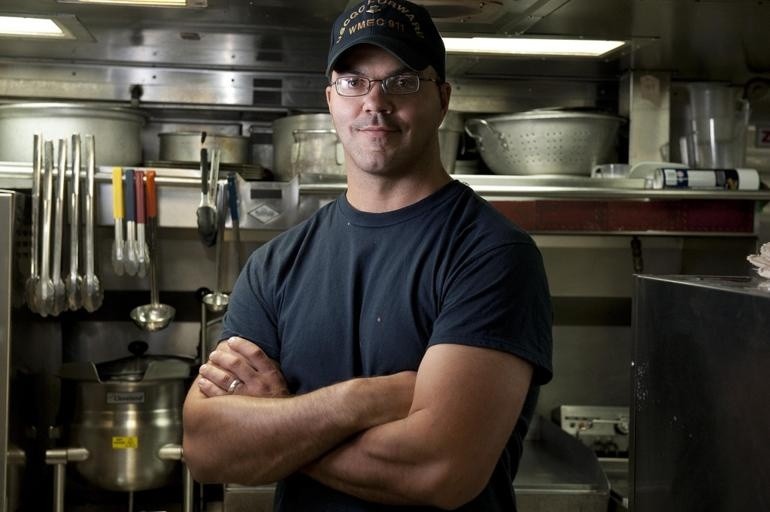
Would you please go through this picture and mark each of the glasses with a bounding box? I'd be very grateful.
[329,74,441,97]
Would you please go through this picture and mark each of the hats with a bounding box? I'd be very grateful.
[325,1,446,79]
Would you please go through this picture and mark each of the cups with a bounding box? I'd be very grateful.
[687,85,752,169]
[601,160,630,180]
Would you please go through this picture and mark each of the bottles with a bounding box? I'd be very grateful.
[653,168,761,192]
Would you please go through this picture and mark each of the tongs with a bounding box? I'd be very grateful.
[25,133,150,317]
[194,146,221,245]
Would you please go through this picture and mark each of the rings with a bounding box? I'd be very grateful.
[228,379,240,392]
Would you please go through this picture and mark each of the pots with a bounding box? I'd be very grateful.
[0,85,627,177]
[68,340,199,493]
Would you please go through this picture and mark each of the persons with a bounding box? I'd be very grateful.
[180,0,555,512]
